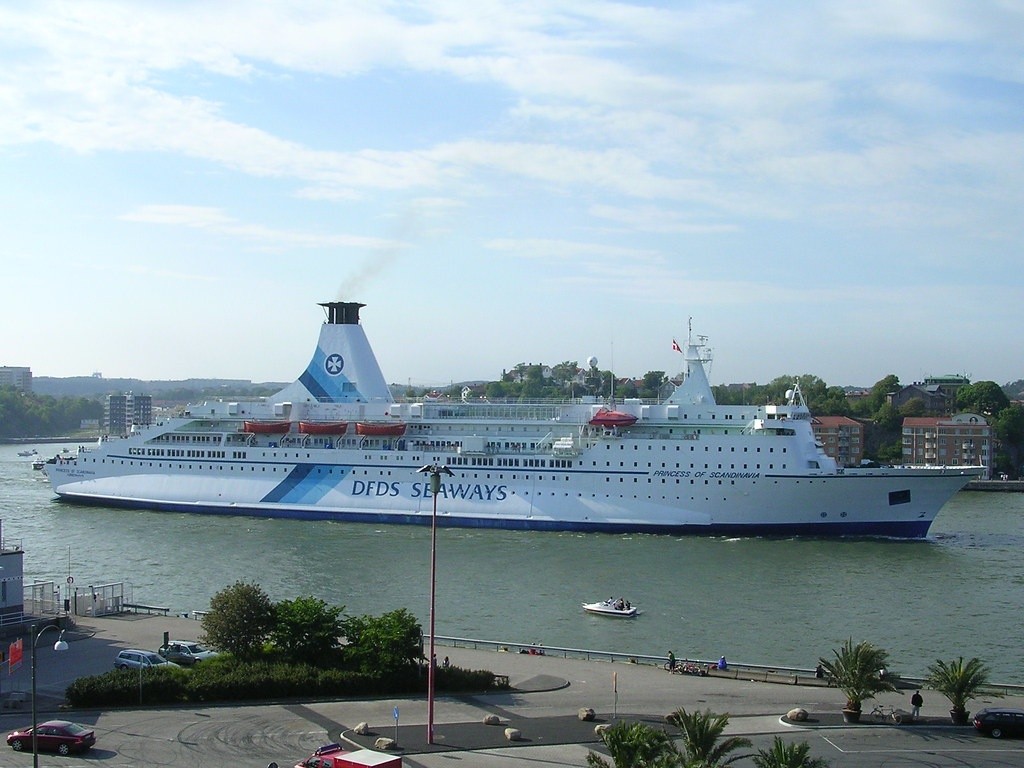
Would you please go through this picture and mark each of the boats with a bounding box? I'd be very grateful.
[18,450,33,456]
[582,595,636,618]
[32,458,46,470]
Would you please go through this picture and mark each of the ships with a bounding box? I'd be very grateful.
[42,302,990,539]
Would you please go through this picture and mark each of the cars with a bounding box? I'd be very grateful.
[114,650,180,671]
[157,641,219,665]
[972,707,1024,738]
[6,714,96,756]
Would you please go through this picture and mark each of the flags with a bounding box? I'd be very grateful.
[672,340,682,353]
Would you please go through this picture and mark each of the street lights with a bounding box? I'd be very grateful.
[26,625,69,768]
[416,465,454,744]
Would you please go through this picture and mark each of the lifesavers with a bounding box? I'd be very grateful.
[67,576,73,584]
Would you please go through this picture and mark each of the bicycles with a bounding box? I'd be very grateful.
[664,660,709,676]
[869,703,902,726]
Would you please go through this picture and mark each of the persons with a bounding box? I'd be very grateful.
[538,643,546,655]
[433,654,437,667]
[815,665,825,678]
[668,650,675,674]
[608,597,612,606]
[620,598,624,610]
[911,689,923,716]
[1001,474,1009,481]
[529,642,538,654]
[623,600,630,610]
[879,668,888,678]
[718,656,727,669]
[442,656,449,669]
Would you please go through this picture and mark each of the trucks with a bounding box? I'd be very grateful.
[294,743,402,768]
